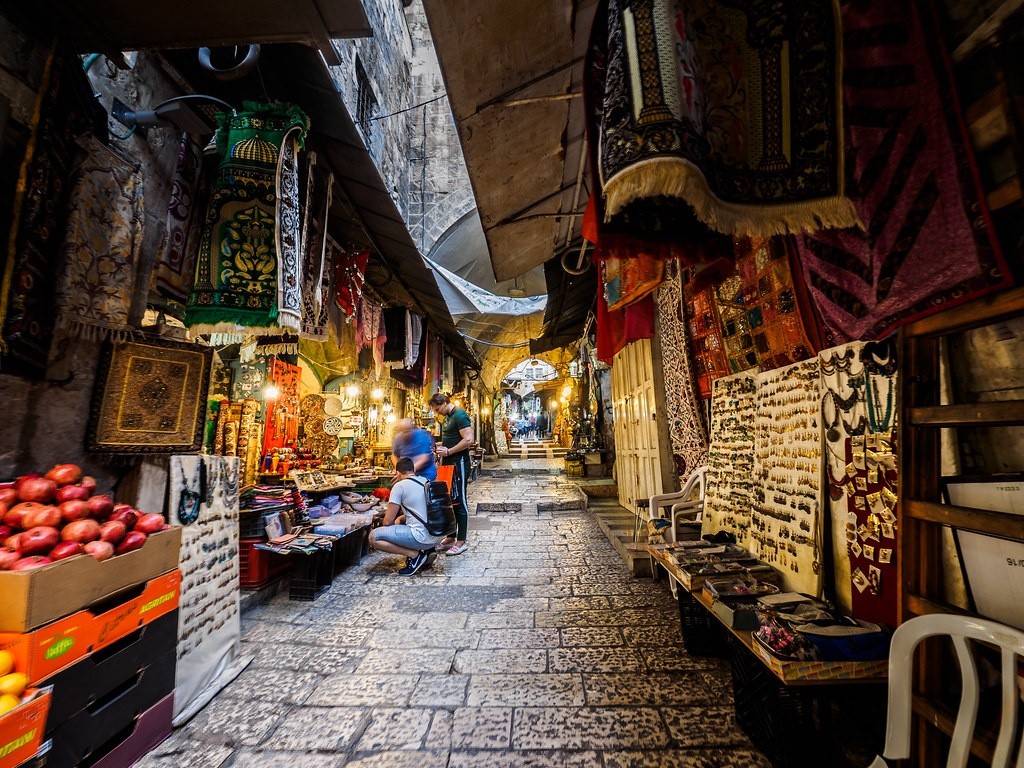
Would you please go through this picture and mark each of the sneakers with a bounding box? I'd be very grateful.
[398,549,427,576]
[423,547,437,567]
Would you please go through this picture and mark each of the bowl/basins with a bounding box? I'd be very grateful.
[341,491,362,503]
[372,506,387,515]
[794,621,882,653]
[361,498,381,506]
[352,502,373,512]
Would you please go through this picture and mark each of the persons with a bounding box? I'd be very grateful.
[368,457,448,576]
[390,417,438,482]
[428,393,474,556]
[508,411,546,439]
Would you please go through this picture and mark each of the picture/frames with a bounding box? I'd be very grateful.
[84,333,214,453]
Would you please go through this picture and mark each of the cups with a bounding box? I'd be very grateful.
[435,442,443,449]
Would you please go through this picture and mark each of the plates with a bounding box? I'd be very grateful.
[324,397,343,415]
[323,417,343,435]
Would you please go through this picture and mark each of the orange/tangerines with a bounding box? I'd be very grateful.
[0,649,14,675]
[0,673,26,696]
[0,695,18,716]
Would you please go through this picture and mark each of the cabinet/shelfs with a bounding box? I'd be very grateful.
[610,339,675,520]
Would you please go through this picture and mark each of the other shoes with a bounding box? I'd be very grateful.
[435,541,455,551]
[446,542,468,556]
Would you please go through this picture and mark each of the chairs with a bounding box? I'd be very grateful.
[649,466,708,600]
[867,614,1024,768]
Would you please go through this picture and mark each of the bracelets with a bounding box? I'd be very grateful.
[447,449,450,456]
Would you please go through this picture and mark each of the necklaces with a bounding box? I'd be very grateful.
[269,359,301,448]
[177,452,241,526]
[818,341,900,489]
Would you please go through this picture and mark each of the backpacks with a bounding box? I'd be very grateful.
[401,478,456,536]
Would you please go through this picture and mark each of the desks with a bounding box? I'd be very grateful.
[645,540,888,685]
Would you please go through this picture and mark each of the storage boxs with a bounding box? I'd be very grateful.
[239,505,369,602]
[731,634,852,768]
[0,526,182,768]
[676,581,729,658]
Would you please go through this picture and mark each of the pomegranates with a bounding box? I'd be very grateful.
[0,463,171,570]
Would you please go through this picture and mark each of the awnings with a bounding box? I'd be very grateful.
[255,37,483,373]
[421,0,601,356]
[0,0,376,74]
